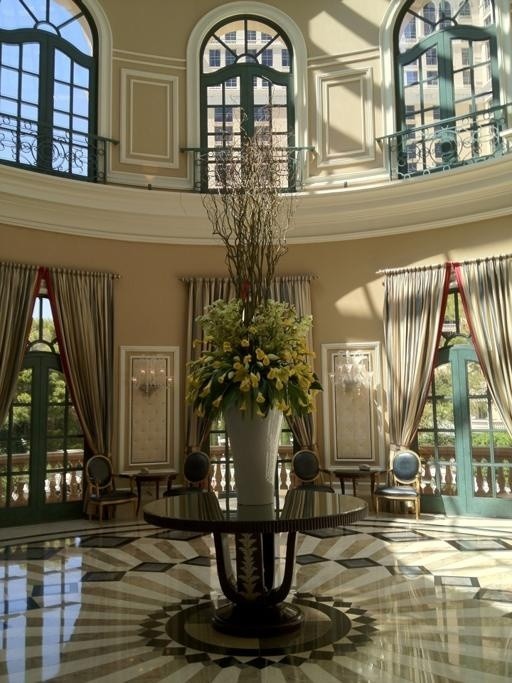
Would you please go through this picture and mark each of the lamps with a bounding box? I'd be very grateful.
[329,349,374,397]
[132,369,173,399]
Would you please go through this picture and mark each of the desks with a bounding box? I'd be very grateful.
[322,464,385,514]
[143,492,368,637]
[119,470,179,516]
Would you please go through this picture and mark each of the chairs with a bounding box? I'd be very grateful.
[292,449,335,493]
[81,454,137,524]
[163,451,212,498]
[375,450,422,520]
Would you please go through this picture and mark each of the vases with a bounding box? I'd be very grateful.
[221,400,287,507]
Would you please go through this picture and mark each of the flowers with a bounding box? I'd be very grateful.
[184,106,322,420]
